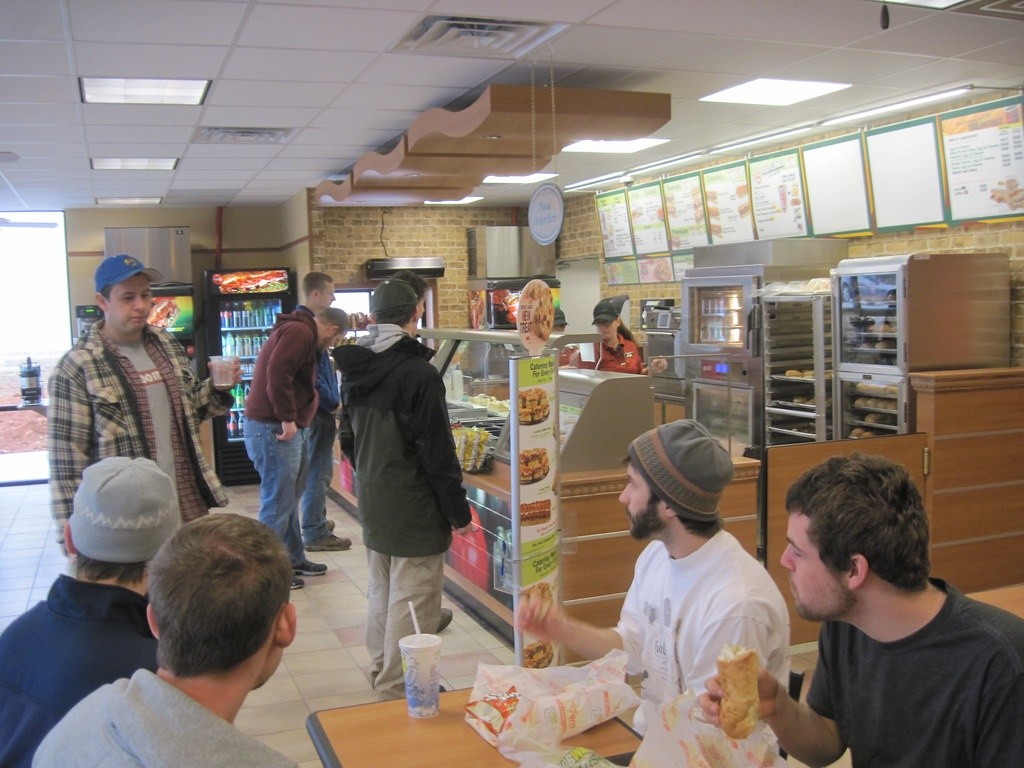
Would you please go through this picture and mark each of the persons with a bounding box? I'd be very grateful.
[0,456,311,768]
[578,293,666,375]
[48,253,246,559]
[244,308,350,588]
[392,267,453,633]
[332,278,477,703]
[301,271,354,552]
[691,449,1024,768]
[550,307,582,365]
[519,420,789,740]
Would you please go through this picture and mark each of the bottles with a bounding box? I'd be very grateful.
[218,300,282,438]
[327,312,370,359]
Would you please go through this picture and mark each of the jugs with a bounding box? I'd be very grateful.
[442,370,463,401]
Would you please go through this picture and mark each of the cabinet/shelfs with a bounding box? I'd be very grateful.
[760,296,830,443]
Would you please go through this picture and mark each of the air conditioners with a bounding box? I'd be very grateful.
[363,256,447,283]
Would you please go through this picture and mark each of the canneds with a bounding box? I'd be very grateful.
[220,305,280,328]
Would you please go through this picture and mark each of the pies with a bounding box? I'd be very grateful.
[716,643,763,740]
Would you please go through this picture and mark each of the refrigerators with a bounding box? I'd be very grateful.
[200,267,298,486]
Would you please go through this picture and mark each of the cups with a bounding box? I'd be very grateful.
[399,634,442,719]
[209,357,235,386]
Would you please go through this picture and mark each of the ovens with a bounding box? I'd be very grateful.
[677,236,1012,558]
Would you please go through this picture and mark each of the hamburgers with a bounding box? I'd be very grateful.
[146,300,181,328]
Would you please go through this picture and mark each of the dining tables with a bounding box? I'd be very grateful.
[306,695,646,768]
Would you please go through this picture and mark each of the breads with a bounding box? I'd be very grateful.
[520,499,551,525]
[785,291,899,438]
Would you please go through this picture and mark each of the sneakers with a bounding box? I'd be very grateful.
[327,521,335,532]
[304,534,352,551]
[291,579,304,590]
[293,559,327,576]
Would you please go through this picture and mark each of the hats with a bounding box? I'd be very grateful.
[95,254,162,293]
[591,300,618,325]
[371,278,418,312]
[69,457,179,563]
[628,419,733,522]
[554,309,568,325]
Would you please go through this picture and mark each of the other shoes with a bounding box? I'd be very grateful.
[436,608,453,633]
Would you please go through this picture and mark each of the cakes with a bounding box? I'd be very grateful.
[520,448,548,481]
[518,388,550,422]
[523,639,554,668]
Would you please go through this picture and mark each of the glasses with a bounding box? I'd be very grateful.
[595,320,613,327]
[554,324,569,328]
